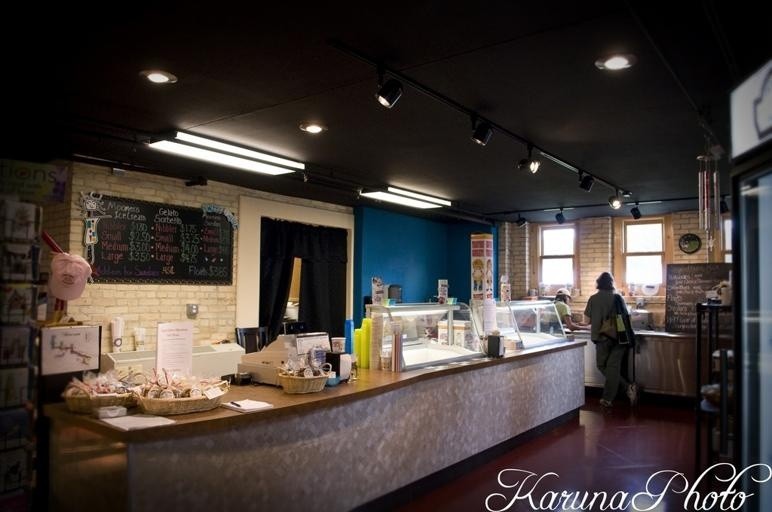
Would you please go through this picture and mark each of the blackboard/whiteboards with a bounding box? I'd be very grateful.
[664,262,732,334]
[81,192,235,286]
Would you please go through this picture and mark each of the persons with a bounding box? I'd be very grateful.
[584,272,640,407]
[542,289,592,335]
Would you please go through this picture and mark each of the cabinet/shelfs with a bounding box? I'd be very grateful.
[690,303,742,481]
[364,299,488,372]
[468,297,569,350]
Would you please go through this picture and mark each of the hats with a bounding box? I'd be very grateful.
[596,272,615,281]
[556,289,572,300]
[50,253,92,301]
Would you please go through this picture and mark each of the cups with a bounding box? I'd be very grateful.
[380,354,392,371]
[110,315,124,353]
[133,326,145,352]
[344,313,384,370]
[331,336,346,353]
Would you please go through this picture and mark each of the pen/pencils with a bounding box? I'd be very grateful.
[230,401,241,407]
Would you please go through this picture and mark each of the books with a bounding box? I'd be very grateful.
[0,201,43,512]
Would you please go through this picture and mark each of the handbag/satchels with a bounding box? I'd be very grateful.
[598,318,618,340]
[615,313,632,344]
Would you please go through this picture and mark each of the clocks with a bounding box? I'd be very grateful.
[678,233,701,254]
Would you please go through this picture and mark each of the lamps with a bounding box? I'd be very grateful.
[147,129,310,185]
[514,205,645,230]
[367,64,632,210]
[359,184,455,212]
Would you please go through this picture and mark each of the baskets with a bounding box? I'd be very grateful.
[61,372,148,413]
[277,363,332,394]
[138,388,229,415]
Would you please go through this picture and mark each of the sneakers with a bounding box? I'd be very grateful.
[627,383,637,405]
[600,399,612,407]
